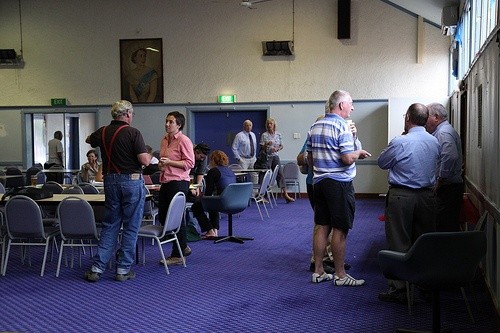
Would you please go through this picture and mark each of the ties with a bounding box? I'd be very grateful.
[248,133,255,156]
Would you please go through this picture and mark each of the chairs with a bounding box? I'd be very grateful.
[0,154,303,279]
[376,231,488,333]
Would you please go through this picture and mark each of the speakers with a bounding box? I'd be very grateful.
[0,49,25,68]
[337,0,358,45]
[262,40,295,61]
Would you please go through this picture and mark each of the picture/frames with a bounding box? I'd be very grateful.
[118,37,165,104]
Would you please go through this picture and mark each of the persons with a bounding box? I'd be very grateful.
[84,99,152,282]
[421,103,464,236]
[47,130,65,182]
[306,89,369,287]
[232,119,258,199]
[190,150,236,241]
[183,141,210,195]
[298,112,362,272]
[377,102,440,302]
[257,117,295,204]
[157,111,194,265]
[80,149,102,182]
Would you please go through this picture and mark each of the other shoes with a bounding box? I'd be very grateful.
[311,271,333,283]
[115,266,136,282]
[83,269,101,282]
[284,193,295,204]
[201,234,219,240]
[309,258,352,274]
[334,274,365,287]
[159,244,192,266]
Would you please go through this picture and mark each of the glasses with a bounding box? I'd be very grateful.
[402,114,411,120]
[129,111,136,118]
